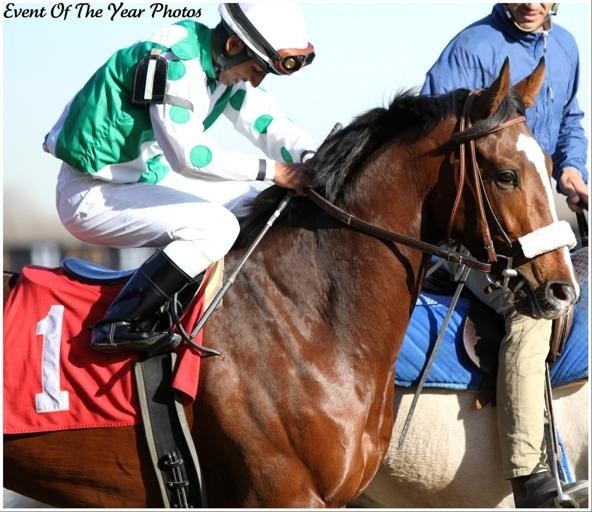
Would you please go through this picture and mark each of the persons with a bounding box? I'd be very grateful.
[418,3,588,508]
[42,3,316,355]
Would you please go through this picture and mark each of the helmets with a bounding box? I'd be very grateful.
[220,2,316,75]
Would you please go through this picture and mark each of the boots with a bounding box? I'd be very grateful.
[511,473,587,509]
[91,248,192,354]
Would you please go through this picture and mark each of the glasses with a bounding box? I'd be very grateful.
[269,43,315,74]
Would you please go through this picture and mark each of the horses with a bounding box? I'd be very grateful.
[342,244,589,509]
[3,54,582,508]
